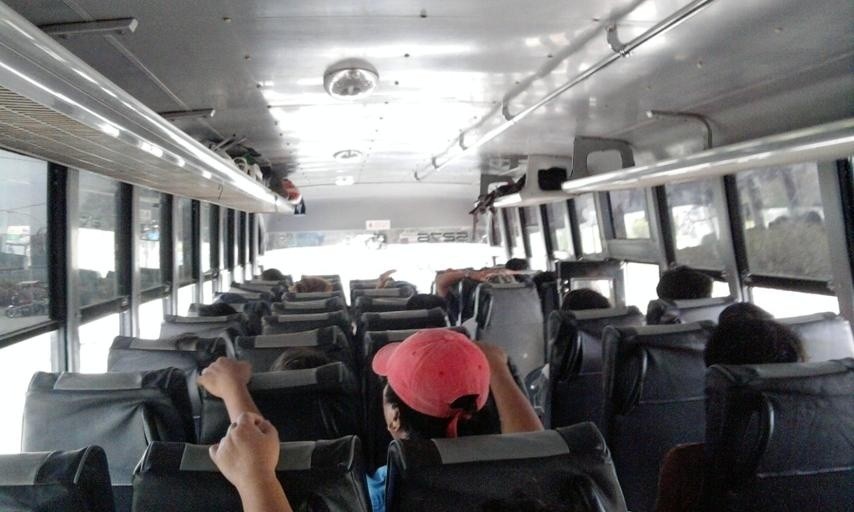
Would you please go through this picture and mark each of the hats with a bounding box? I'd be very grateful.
[372,328,491,420]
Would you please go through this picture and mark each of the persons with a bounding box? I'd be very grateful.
[195,329,542,512]
[655,261,715,301]
[207,411,294,511]
[717,301,775,325]
[534,287,611,402]
[265,349,326,371]
[504,258,531,283]
[649,320,801,511]
[435,263,542,326]
[287,277,332,294]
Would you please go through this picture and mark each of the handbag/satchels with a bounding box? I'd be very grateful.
[204,137,299,200]
[468,167,567,216]
